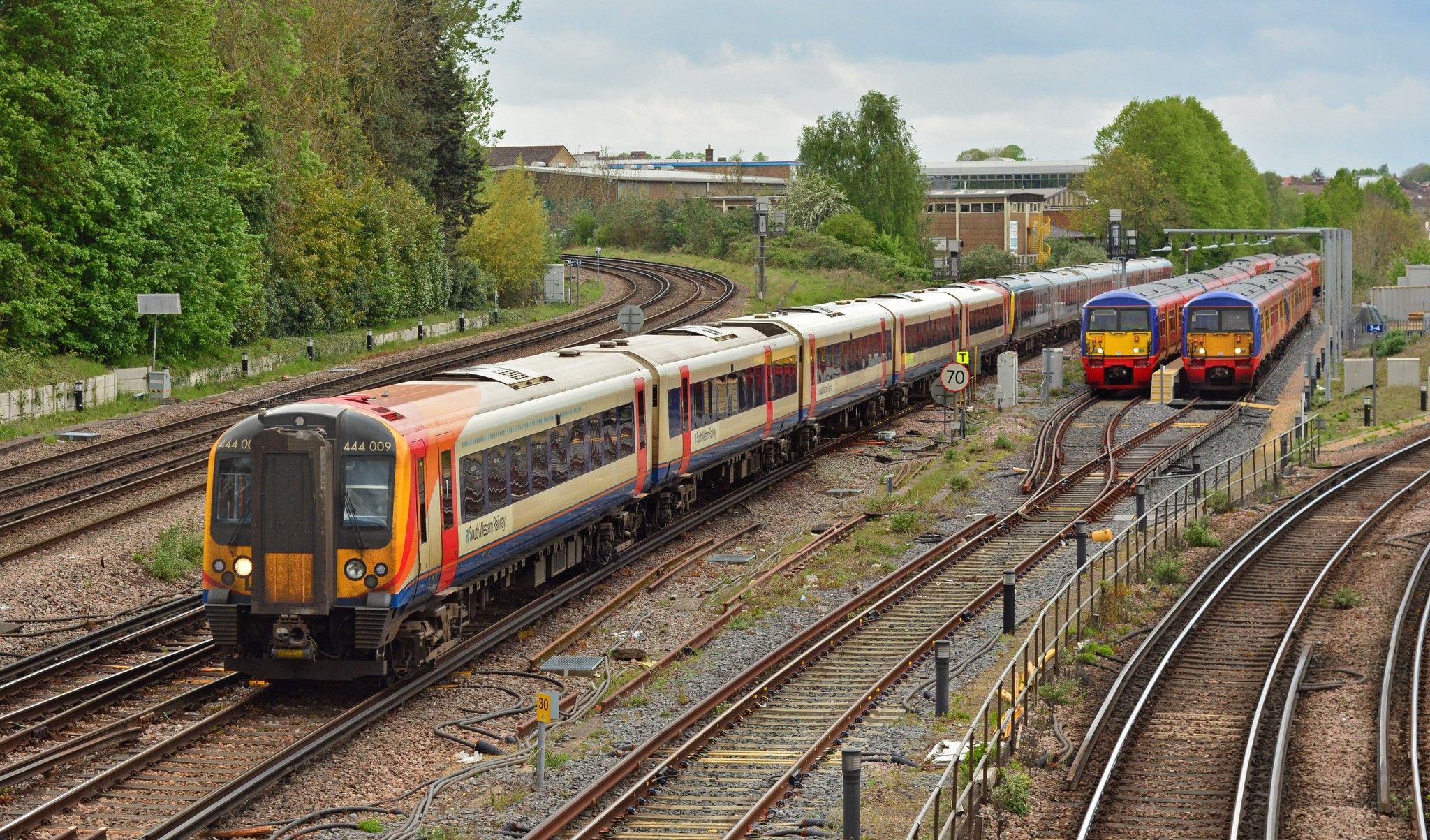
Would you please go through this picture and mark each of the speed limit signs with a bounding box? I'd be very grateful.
[940,363,969,392]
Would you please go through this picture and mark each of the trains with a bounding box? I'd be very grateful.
[203,256,1173,686]
[1080,251,1325,398]
